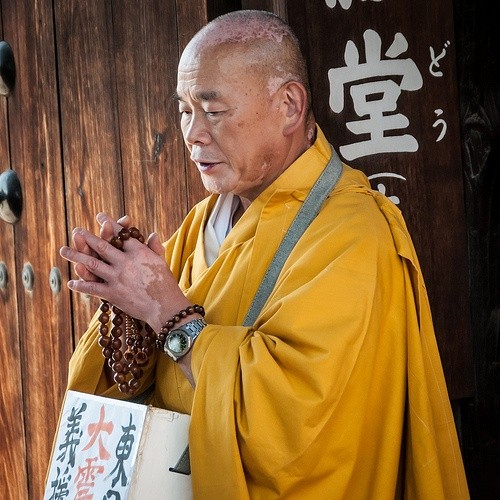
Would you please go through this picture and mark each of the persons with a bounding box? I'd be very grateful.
[44,10,470,500]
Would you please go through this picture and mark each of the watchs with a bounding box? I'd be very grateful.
[163,318,204,361]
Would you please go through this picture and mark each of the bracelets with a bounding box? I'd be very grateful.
[156,305,205,351]
